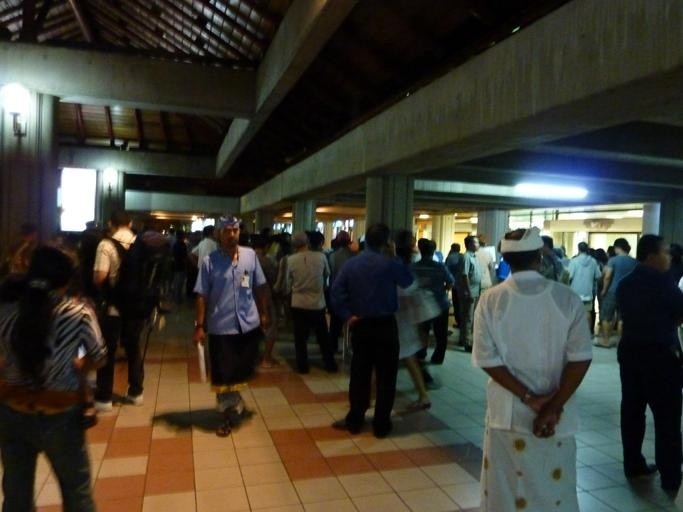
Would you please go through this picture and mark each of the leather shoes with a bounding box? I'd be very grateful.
[624,464,658,479]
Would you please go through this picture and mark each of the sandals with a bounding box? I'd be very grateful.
[406,400,430,411]
[216,420,231,437]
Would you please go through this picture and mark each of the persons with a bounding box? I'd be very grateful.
[542,236,633,347]
[241,224,432,437]
[1,224,108,512]
[474,225,591,511]
[415,236,511,361]
[82,210,265,435]
[615,231,683,490]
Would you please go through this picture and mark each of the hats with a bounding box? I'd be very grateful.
[336,231,350,240]
[213,215,242,240]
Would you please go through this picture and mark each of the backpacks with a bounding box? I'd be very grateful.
[112,238,161,322]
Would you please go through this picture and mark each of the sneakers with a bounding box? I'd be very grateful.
[121,391,144,406]
[91,400,113,412]
[258,354,282,367]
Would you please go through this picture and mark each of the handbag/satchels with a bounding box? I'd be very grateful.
[400,291,442,326]
